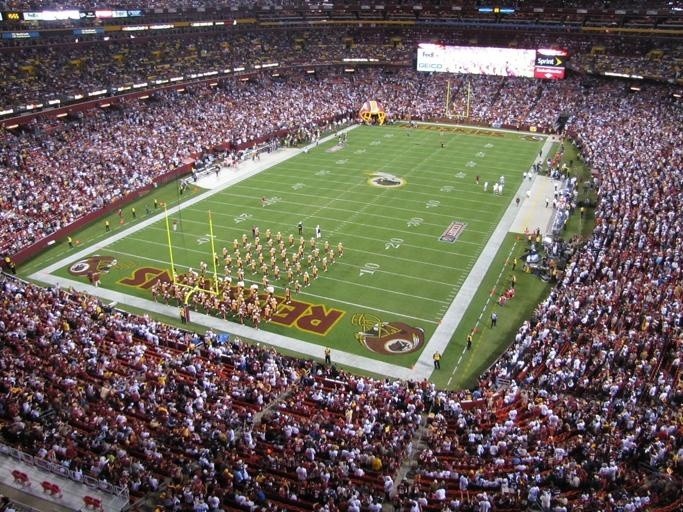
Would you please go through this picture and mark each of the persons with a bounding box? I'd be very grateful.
[483,176,505,196]
[338,1,518,128]
[1,269,424,512]
[199,226,342,272]
[491,311,497,327]
[153,1,342,192]
[172,218,179,232]
[424,351,516,509]
[476,176,479,184]
[516,197,520,207]
[297,221,302,235]
[521,1,682,511]
[466,334,472,349]
[496,273,516,306]
[0,0,153,269]
[315,225,320,238]
[512,257,516,270]
[153,197,158,210]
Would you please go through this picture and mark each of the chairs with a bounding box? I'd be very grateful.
[101,337,682,512]
